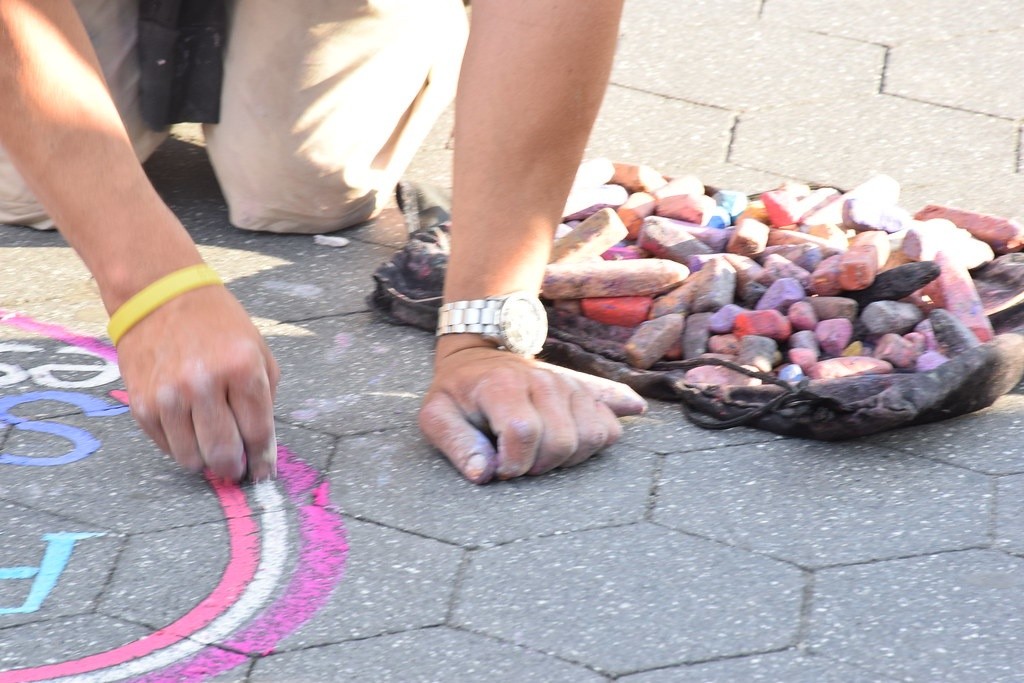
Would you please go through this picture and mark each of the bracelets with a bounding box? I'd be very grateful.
[107,262,224,346]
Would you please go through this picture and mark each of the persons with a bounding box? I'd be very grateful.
[0,0,648,484]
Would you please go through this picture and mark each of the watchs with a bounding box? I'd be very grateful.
[434,290,549,356]
